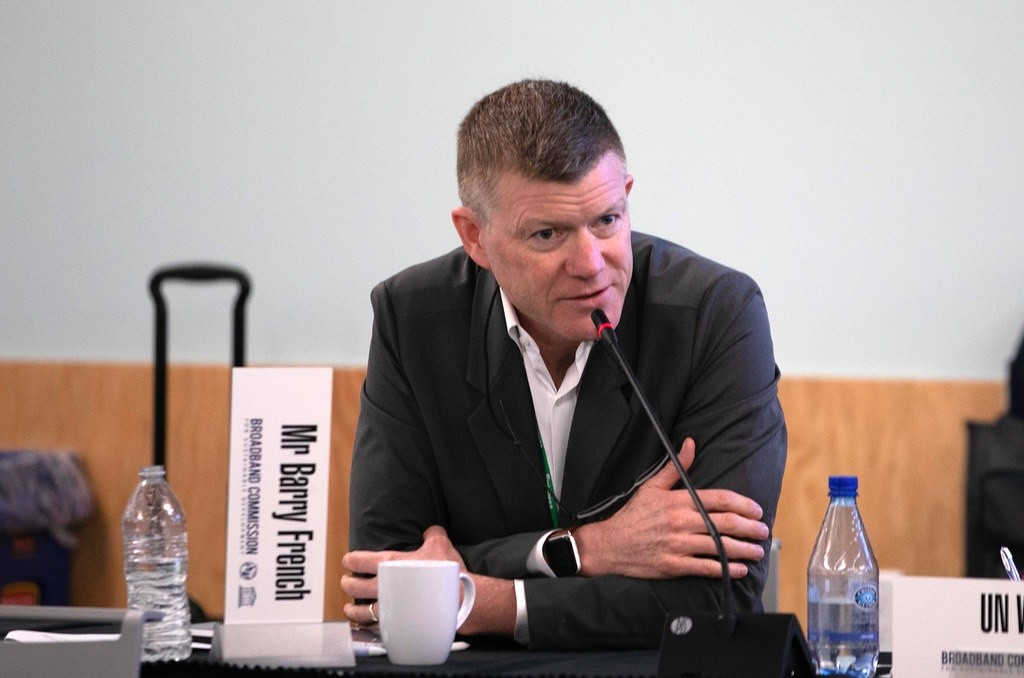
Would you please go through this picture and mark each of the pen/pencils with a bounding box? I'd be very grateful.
[998,545,1019,580]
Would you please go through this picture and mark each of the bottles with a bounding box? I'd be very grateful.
[120,465,193,662]
[807,476,880,678]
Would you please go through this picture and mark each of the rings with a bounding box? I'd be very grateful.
[369,604,378,622]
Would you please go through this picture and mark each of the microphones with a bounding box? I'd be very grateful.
[590,307,816,678]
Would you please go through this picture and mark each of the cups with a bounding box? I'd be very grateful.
[377,559,476,666]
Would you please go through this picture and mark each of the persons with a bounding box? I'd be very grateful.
[340,80,788,645]
[967,338,1024,572]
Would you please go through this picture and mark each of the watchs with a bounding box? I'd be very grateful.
[545,526,582,578]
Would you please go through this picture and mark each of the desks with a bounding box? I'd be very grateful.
[42,621,892,678]
